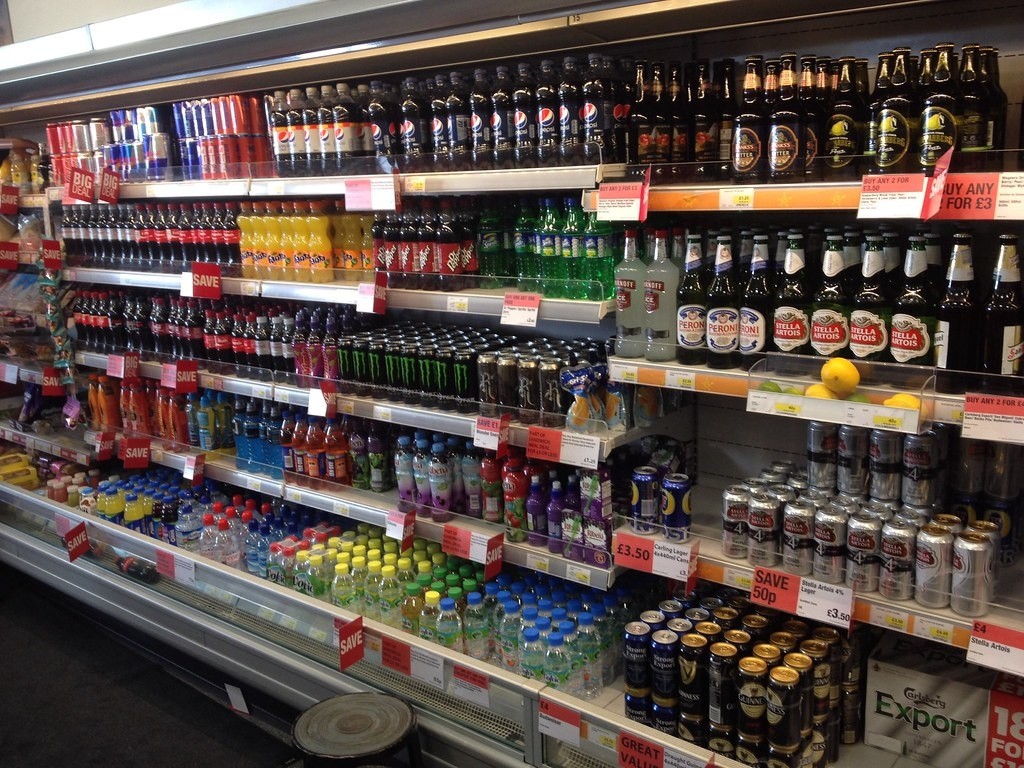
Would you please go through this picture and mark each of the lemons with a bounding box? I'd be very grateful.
[756,357,929,426]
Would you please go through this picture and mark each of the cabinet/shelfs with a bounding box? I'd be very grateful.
[2,2,1024,768]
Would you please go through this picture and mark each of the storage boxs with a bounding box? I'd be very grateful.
[863,631,1024,768]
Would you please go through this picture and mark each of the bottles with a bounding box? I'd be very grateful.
[47,42,1024,701]
[1,143,54,195]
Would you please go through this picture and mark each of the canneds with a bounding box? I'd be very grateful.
[44,94,280,186]
[334,320,619,429]
[630,418,1024,617]
[621,582,870,768]
